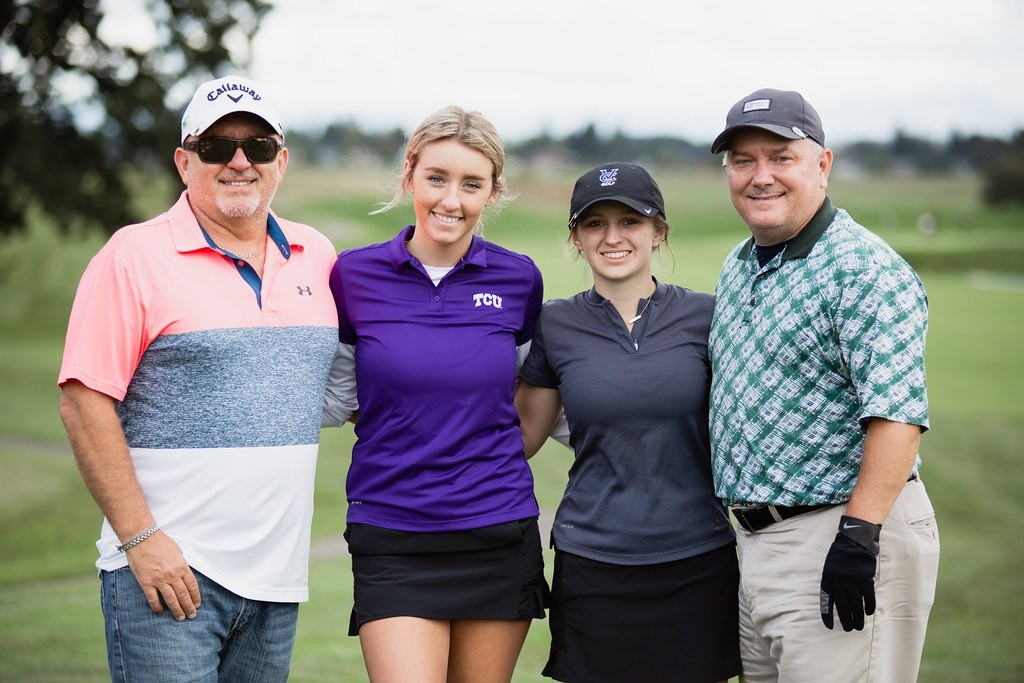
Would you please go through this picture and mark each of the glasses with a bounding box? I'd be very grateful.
[182,134,284,165]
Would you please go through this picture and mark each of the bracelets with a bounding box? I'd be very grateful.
[116,523,158,554]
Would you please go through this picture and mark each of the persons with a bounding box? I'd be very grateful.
[712,89,940,683]
[319,106,574,683]
[57,78,360,682]
[513,163,742,683]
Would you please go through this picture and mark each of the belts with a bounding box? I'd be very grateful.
[732,476,914,534]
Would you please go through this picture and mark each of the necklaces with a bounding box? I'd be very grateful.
[629,294,652,323]
[219,244,267,258]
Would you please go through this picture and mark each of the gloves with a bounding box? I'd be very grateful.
[821,515,883,632]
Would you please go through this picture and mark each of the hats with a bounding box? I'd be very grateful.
[712,88,825,155]
[178,75,285,150]
[569,162,665,227]
